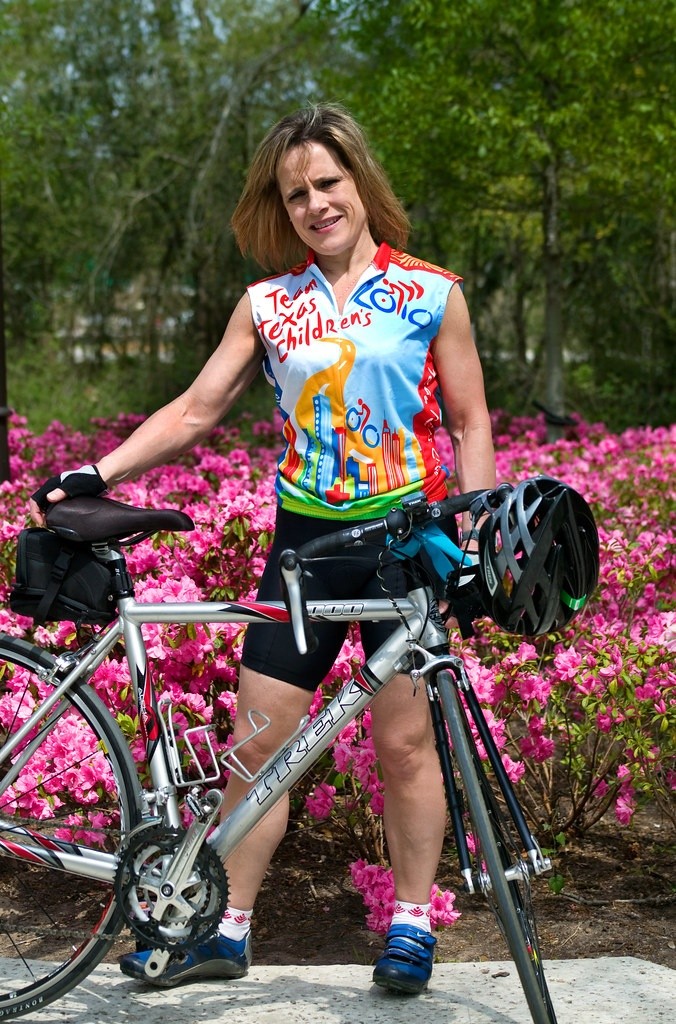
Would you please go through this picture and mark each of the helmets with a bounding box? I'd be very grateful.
[470,475,599,637]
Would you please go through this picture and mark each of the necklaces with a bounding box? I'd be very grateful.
[337,260,372,303]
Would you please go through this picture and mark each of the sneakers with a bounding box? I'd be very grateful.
[119,925,254,986]
[372,923,437,993]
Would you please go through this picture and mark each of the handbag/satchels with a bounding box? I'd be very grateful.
[8,526,118,627]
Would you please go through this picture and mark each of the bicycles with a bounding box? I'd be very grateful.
[0,480,558,1024]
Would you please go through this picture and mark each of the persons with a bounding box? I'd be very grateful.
[23,100,494,994]
